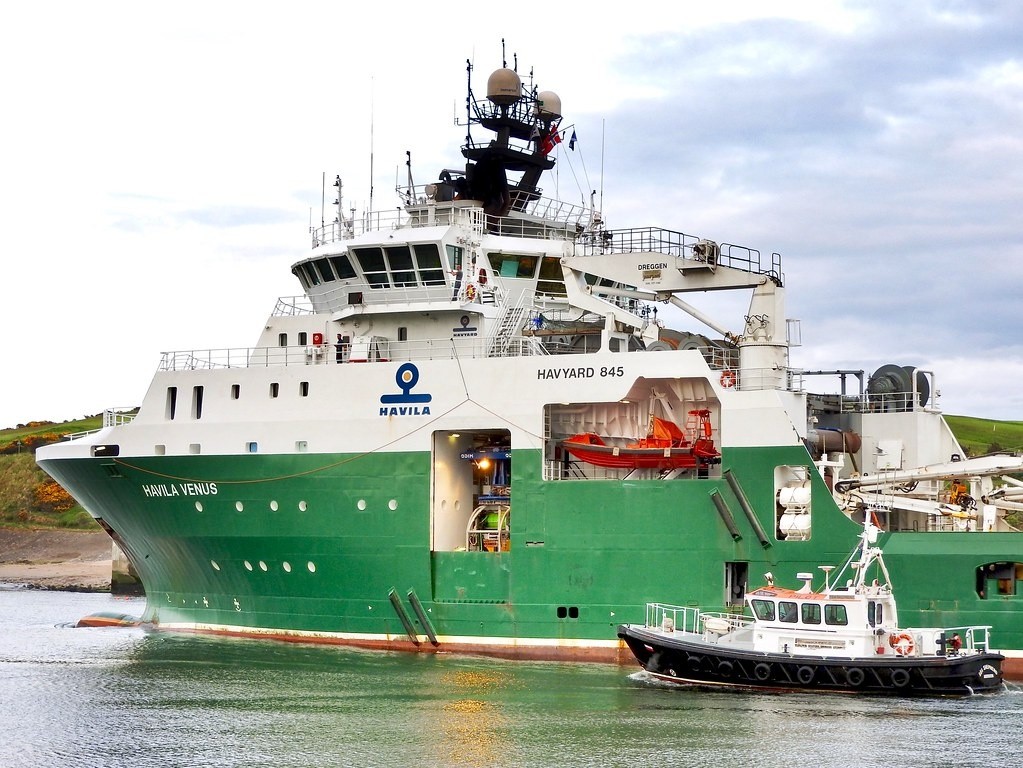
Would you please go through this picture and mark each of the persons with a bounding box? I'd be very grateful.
[334,334,344,364]
[451,264,463,301]
[782,604,798,622]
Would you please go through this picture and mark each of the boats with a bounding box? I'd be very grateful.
[610,502,1008,696]
[36,27,1022,669]
[564,411,719,468]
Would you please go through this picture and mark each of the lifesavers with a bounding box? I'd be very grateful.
[894,634,913,655]
[466,284,475,300]
[891,669,910,687]
[479,268,487,286]
[717,661,734,678]
[797,666,815,685]
[720,371,735,387]
[847,667,865,686]
[686,656,702,673]
[754,662,771,681]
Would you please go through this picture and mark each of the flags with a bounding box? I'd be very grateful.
[540,125,561,157]
[569,130,577,150]
